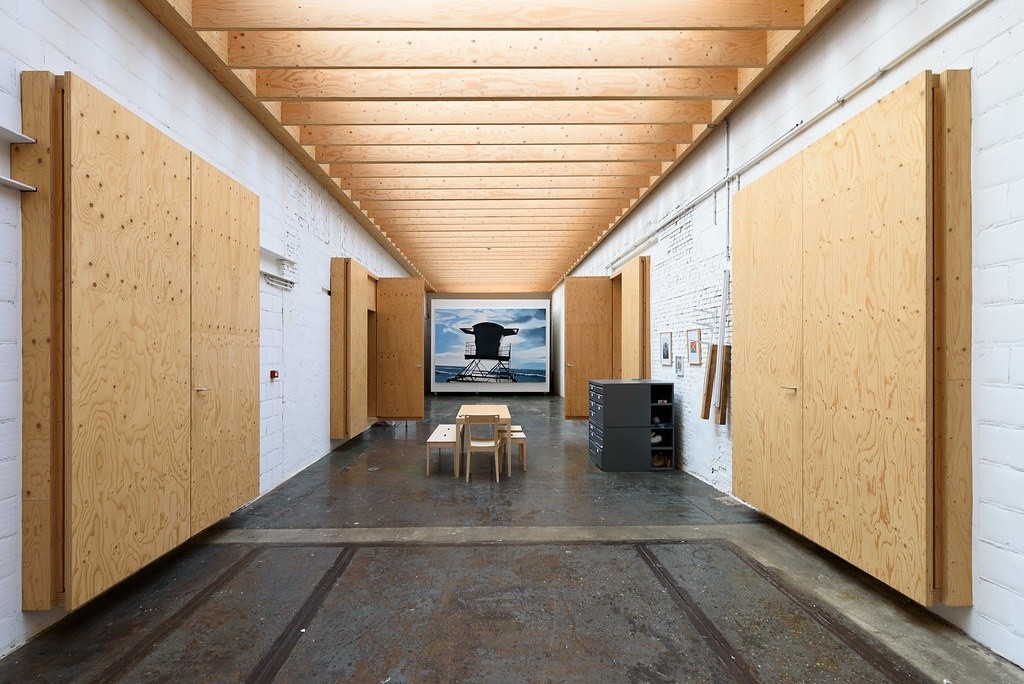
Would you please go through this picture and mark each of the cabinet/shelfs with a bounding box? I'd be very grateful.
[588,418,674,472]
[731,67,971,609]
[10,69,260,613]
[563,255,651,419]
[329,256,425,442]
[589,379,674,428]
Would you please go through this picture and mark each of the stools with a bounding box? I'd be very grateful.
[498,432,527,472]
[499,425,522,462]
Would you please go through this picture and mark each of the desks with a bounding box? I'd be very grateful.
[455,404,511,478]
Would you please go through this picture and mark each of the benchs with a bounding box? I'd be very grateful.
[426,424,463,477]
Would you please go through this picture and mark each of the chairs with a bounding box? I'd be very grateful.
[465,414,502,483]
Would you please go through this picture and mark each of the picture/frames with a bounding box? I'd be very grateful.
[660,332,672,367]
[674,355,684,377]
[686,328,702,365]
